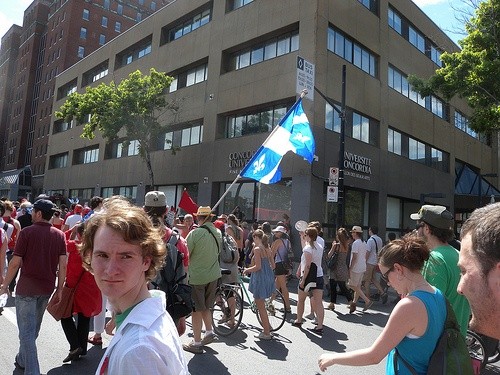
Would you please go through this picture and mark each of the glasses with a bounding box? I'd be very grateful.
[415,223,425,230]
[381,266,394,282]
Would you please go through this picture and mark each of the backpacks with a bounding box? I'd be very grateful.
[393,295,474,375]
[147,230,194,320]
[222,234,239,263]
[277,238,295,271]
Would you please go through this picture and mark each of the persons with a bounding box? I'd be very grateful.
[410,201,472,343]
[76,195,192,375]
[1,193,106,301]
[63,222,104,362]
[181,206,222,354]
[456,201,500,341]
[166,206,416,336]
[2,199,68,375]
[318,235,449,375]
[106,191,191,336]
[87,294,107,345]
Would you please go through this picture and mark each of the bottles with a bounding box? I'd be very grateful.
[0,285,10,307]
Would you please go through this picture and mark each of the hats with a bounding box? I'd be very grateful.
[410,204,452,230]
[193,205,216,216]
[349,226,363,233]
[21,201,32,208]
[217,213,227,219]
[74,204,83,213]
[272,226,286,233]
[81,207,92,214]
[144,191,167,207]
[32,198,54,211]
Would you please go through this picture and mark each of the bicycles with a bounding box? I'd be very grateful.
[474,331,499,363]
[209,267,244,337]
[212,266,287,332]
[466,330,488,373]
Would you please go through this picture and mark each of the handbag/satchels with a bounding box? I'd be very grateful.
[47,279,77,322]
[327,243,341,268]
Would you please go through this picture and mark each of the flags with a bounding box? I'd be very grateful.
[239,97,318,186]
[179,190,200,220]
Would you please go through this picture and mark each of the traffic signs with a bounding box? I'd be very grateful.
[296,56,316,102]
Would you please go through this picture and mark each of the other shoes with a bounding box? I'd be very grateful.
[15,336,103,375]
[182,274,401,354]
